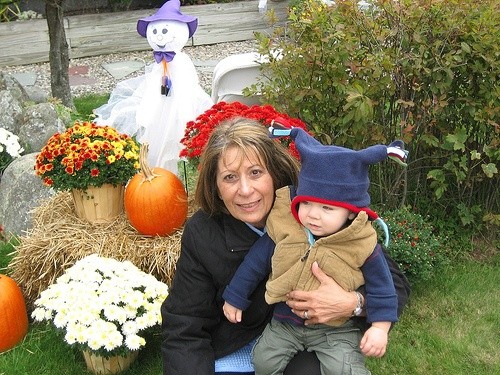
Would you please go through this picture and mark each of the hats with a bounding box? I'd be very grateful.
[269,127,404,225]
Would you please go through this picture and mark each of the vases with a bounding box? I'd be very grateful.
[82,350,139,375]
[71,183,125,224]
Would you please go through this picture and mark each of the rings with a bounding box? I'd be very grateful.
[304,311,310,319]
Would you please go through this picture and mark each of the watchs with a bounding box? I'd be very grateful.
[351,290,364,316]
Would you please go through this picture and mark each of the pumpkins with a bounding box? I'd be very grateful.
[125,144,188,237]
[0,274,29,352]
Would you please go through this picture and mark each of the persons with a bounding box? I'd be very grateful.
[222,126,410,375]
[160,119,409,375]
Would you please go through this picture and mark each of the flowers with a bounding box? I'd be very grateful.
[33,120,141,201]
[30,254,169,361]
[0,128,31,173]
[180,101,314,161]
